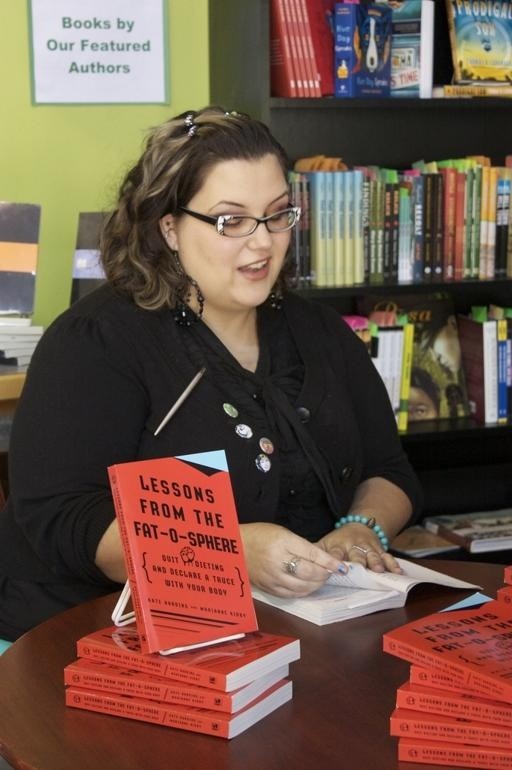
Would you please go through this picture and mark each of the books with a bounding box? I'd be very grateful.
[74,624,305,692]
[107,445,262,653]
[2,306,49,371]
[380,590,511,768]
[389,513,511,563]
[49,680,294,739]
[351,305,512,432]
[270,1,512,98]
[62,656,290,716]
[249,551,485,628]
[279,156,511,284]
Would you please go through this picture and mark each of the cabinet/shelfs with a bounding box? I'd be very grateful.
[204,0,512,527]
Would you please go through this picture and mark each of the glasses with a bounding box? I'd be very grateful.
[176,205,302,239]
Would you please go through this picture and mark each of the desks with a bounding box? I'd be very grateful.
[1,553,510,766]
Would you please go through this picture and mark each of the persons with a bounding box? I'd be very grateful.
[1,102,429,655]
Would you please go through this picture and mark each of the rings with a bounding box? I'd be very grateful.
[287,558,302,576]
